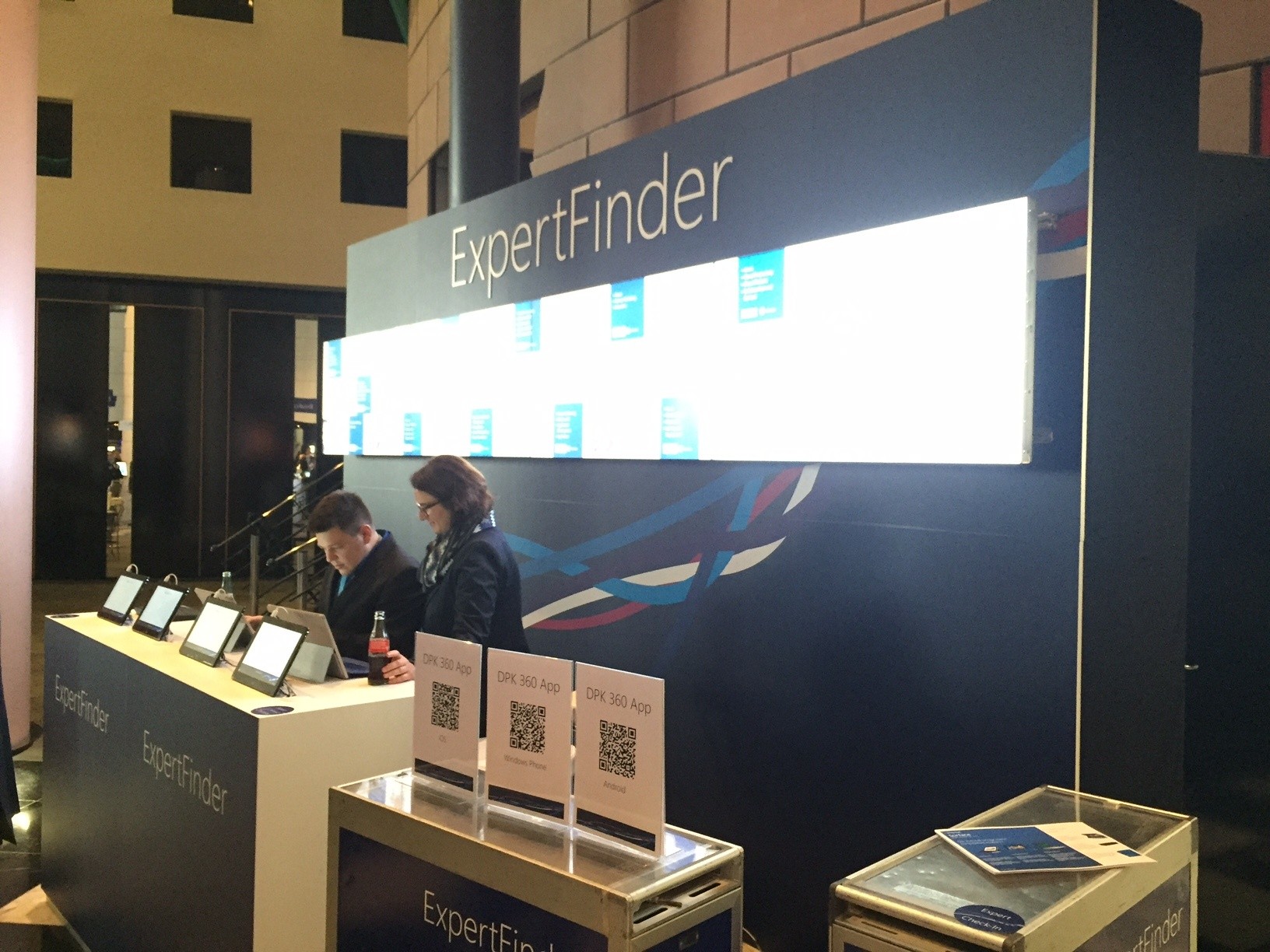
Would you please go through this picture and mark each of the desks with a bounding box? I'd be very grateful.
[38,604,417,952]
[829,785,1199,952]
[325,758,752,951]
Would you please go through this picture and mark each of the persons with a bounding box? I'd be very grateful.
[244,486,423,658]
[383,454,534,738]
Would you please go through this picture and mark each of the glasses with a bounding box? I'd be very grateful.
[417,499,442,517]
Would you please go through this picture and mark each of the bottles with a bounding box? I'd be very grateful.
[368,610,391,686]
[222,571,233,594]
